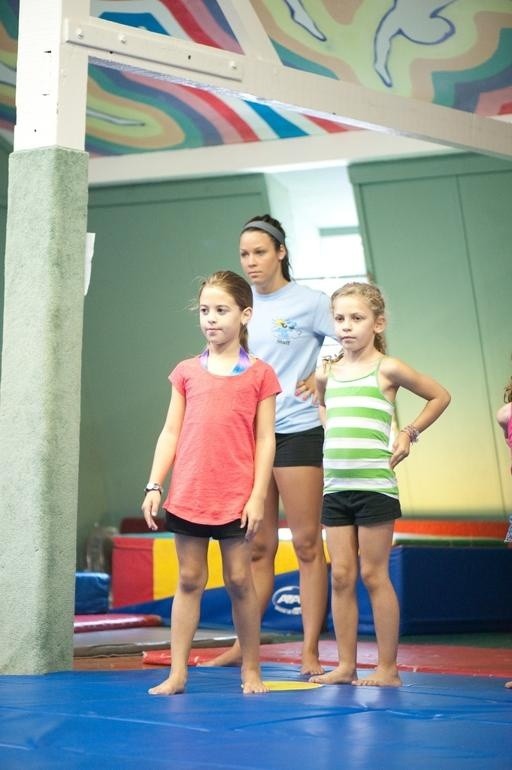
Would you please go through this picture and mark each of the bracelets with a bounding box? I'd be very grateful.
[401,424,419,443]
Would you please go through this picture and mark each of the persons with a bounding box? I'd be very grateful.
[307,281,452,689]
[194,214,340,676]
[495,377,512,693]
[141,269,284,696]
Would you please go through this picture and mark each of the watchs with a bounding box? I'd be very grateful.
[144,481,163,497]
